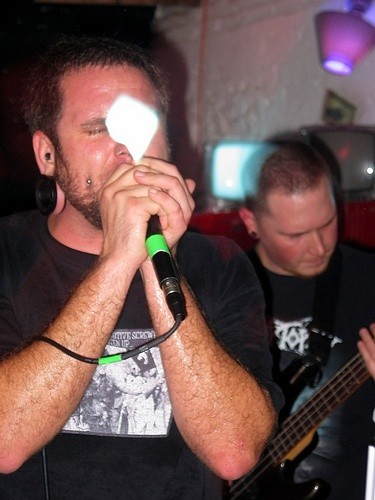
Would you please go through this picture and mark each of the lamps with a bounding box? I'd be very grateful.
[314,0,375,76]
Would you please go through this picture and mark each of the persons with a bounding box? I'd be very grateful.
[238,134,375,500]
[0,36,277,500]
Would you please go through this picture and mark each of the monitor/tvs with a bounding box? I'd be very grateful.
[303,125,375,201]
[202,137,264,210]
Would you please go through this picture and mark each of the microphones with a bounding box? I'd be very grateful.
[146,214,186,321]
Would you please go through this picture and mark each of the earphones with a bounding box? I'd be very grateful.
[45,153,50,160]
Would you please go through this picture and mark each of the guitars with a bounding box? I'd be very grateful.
[222,335,375,500]
